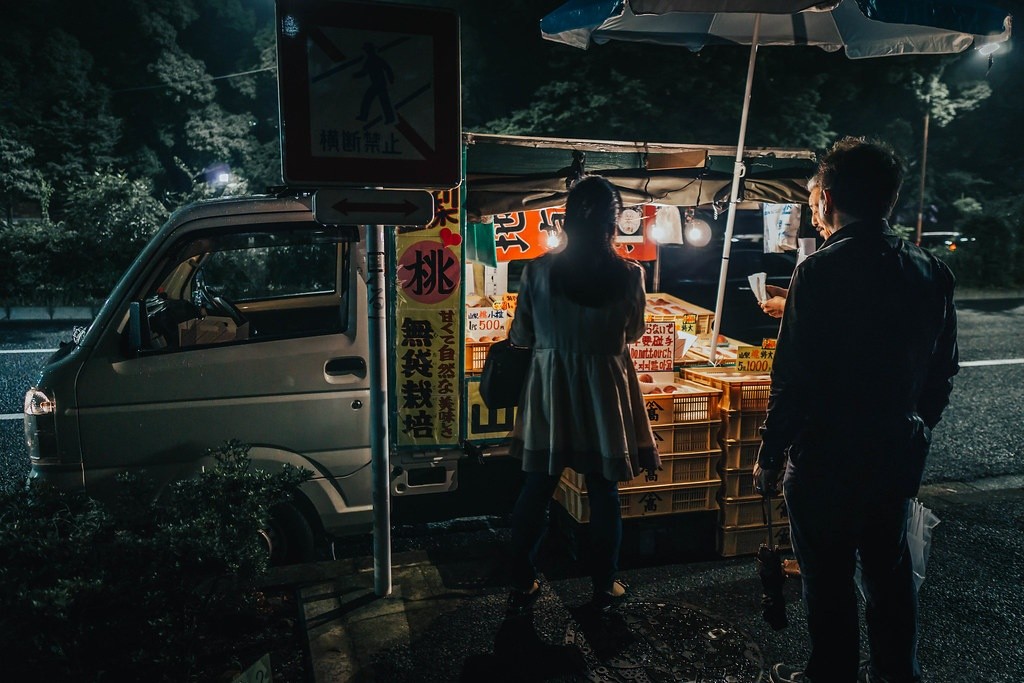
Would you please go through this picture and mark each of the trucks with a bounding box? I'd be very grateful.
[26,170,836,581]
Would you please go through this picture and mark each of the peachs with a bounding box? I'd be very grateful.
[638,374,677,396]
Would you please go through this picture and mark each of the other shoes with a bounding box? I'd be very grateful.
[594,578,630,605]
[506,580,543,609]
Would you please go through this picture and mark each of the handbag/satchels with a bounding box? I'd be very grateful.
[478,338,528,406]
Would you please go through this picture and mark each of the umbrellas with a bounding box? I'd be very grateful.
[539,1,1014,370]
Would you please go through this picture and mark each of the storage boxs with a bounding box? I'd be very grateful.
[461,293,795,559]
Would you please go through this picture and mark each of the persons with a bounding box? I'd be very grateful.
[750,135,962,683]
[756,169,838,320]
[504,175,665,613]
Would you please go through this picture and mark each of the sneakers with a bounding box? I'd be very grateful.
[770,664,809,683]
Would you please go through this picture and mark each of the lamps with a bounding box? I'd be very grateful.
[539,226,569,255]
[684,215,712,248]
[644,217,671,246]
